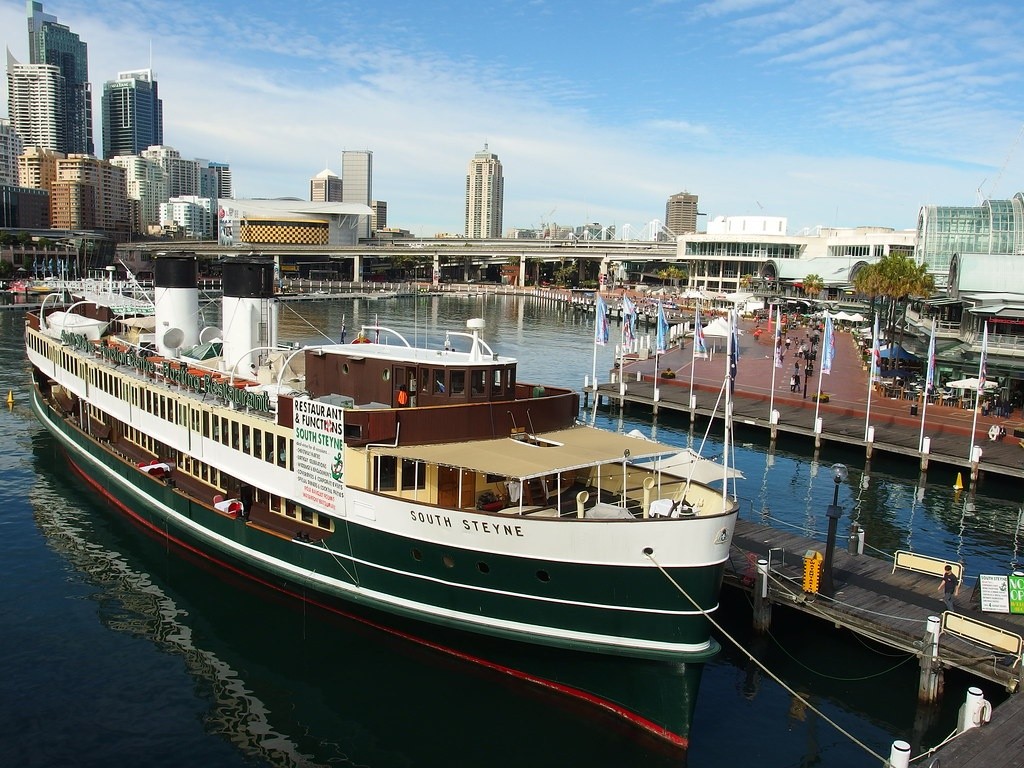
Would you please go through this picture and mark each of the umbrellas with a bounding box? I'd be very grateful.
[821,308,870,326]
[623,428,746,490]
[946,377,998,406]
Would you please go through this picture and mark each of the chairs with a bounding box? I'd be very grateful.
[228,502,239,518]
[150,460,159,465]
[138,462,147,468]
[148,468,165,478]
[213,495,223,504]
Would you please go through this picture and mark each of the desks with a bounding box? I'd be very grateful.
[496,506,558,518]
[214,498,244,516]
[648,499,693,519]
[585,502,637,519]
[139,463,170,473]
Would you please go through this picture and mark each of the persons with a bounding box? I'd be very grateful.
[397,385,408,407]
[937,564,960,612]
[756,313,823,394]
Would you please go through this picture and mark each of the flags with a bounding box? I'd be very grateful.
[597,294,609,346]
[374,314,380,344]
[657,299,669,356]
[340,314,347,344]
[773,304,783,369]
[33,259,77,276]
[871,312,882,383]
[729,310,740,394]
[692,302,707,355]
[621,295,635,350]
[820,311,836,376]
[925,324,936,391]
[977,324,987,394]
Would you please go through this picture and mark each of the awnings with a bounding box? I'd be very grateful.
[372,425,686,517]
[861,328,921,380]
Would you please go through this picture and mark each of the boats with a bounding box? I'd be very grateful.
[22,250,740,751]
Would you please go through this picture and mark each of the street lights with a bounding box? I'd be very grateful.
[801,320,822,399]
[818,461,848,603]
